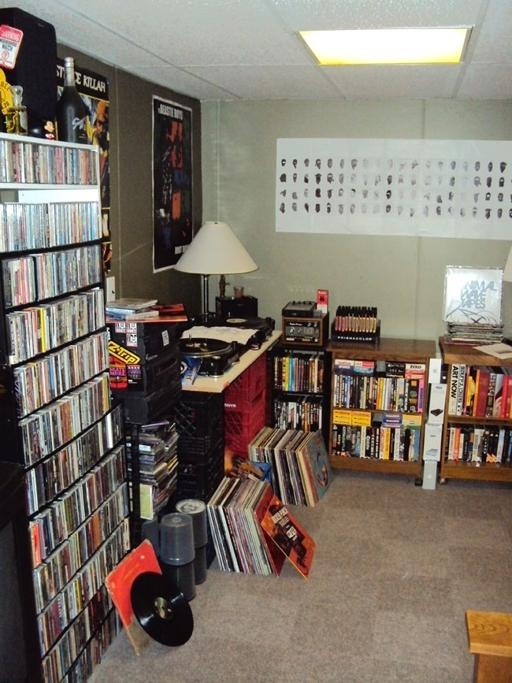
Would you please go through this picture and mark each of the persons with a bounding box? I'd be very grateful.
[154,121,184,249]
[86,101,109,177]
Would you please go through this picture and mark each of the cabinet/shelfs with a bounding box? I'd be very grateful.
[0,132,133,673]
[277,349,326,430]
[327,337,437,485]
[437,334,512,486]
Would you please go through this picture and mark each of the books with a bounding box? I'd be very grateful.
[206,476,317,580]
[249,428,334,508]
[29,445,129,568]
[0,204,101,252]
[0,138,99,184]
[30,481,131,683]
[444,323,512,361]
[125,417,182,521]
[334,359,425,414]
[448,364,512,419]
[13,333,110,419]
[6,287,104,366]
[275,351,322,430]
[3,245,101,308]
[444,424,512,466]
[332,425,420,462]
[105,296,188,322]
[21,371,109,471]
[25,407,124,518]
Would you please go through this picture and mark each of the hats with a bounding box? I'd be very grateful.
[171,121,183,141]
[96,100,108,122]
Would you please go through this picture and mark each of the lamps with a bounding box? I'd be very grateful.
[174,220,259,315]
[293,24,476,68]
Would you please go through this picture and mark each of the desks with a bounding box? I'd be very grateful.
[180,324,283,492]
